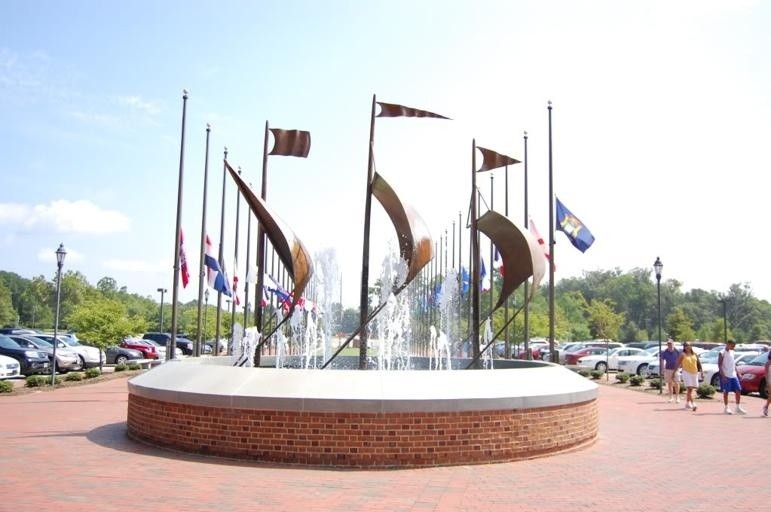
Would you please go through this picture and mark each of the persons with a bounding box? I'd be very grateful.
[662,341,683,403]
[673,341,704,411]
[763,351,771,416]
[718,340,747,414]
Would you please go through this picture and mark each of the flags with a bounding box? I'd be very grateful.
[222,256,318,322]
[204,235,230,296]
[180,228,189,288]
[556,198,595,254]
[421,221,555,310]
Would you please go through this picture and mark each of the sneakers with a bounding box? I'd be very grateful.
[735,409,746,415]
[693,406,696,411]
[724,408,732,415]
[687,404,693,408]
[677,400,680,403]
[763,407,768,416]
[669,399,673,403]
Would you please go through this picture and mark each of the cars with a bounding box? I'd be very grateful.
[0,322,276,385]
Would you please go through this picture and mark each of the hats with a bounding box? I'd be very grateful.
[667,339,673,344]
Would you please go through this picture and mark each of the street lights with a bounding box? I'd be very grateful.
[247,300,252,330]
[651,255,669,393]
[512,294,521,358]
[226,297,232,312]
[157,286,170,333]
[203,287,211,352]
[717,298,734,343]
[49,242,69,385]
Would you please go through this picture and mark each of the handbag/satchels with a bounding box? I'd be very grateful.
[697,360,701,372]
